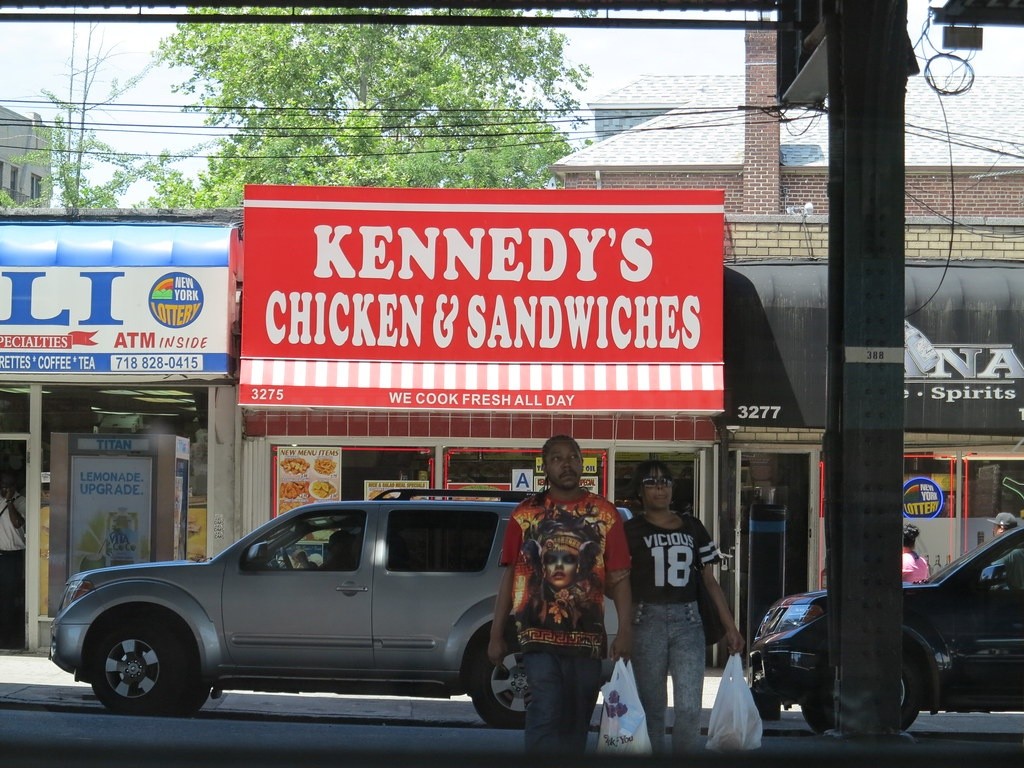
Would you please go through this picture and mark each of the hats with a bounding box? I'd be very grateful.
[987,513,1017,530]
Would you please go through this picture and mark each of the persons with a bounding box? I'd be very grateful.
[0,467,26,646]
[310,530,357,571]
[905,523,928,582]
[488,434,634,752]
[986,512,1024,589]
[605,459,746,754]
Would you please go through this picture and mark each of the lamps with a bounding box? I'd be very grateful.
[801,202,816,258]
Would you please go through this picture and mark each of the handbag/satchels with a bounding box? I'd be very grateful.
[683,510,734,646]
[596,655,652,757]
[705,651,762,753]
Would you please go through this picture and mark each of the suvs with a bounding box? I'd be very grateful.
[48,488,634,729]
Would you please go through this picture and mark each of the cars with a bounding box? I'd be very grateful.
[748,527,1024,736]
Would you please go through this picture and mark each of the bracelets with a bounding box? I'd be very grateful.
[6,499,11,503]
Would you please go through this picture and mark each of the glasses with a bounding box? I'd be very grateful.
[641,478,672,488]
